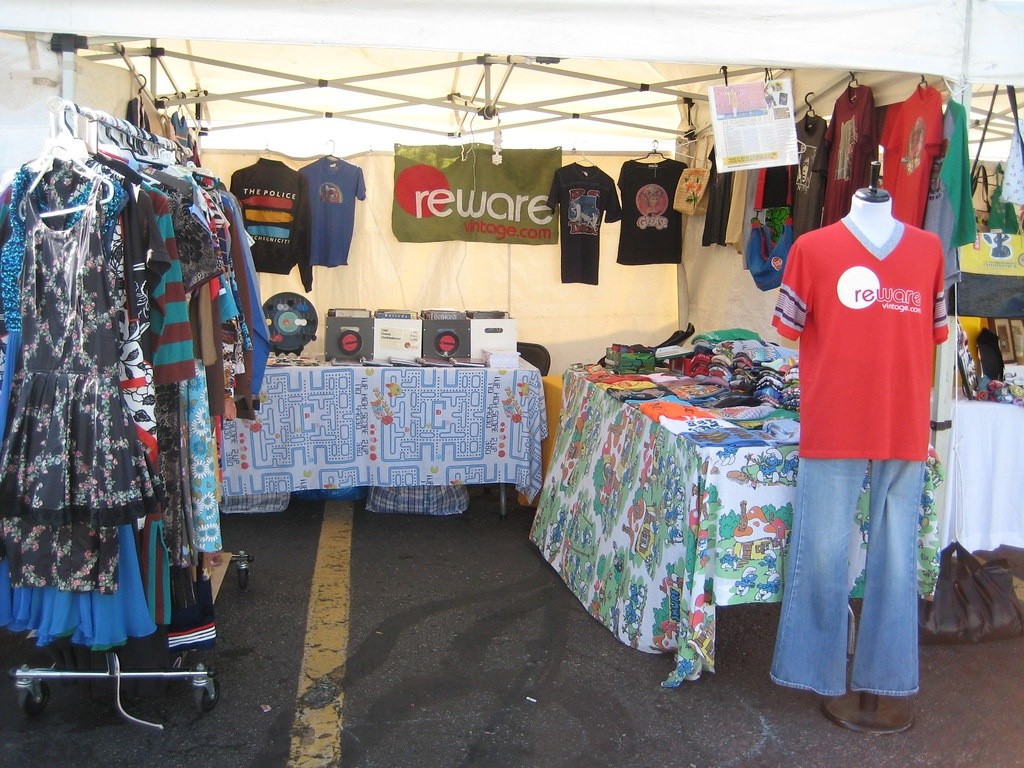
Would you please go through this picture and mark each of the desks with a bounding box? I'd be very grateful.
[517,363,946,688]
[947,386,1024,553]
[222,355,550,521]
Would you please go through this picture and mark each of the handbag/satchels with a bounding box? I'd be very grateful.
[672,138,713,216]
[947,232,1024,318]
[920,540,1023,646]
[746,216,793,291]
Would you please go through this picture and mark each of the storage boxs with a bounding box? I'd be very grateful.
[466,317,516,359]
[418,315,471,359]
[324,313,374,362]
[372,315,422,361]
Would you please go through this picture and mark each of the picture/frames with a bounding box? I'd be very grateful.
[987,318,1018,364]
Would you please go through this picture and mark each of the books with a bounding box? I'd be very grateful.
[359,356,394,367]
[390,355,421,368]
[449,357,486,368]
[329,357,368,366]
[415,355,453,367]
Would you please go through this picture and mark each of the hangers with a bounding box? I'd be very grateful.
[976,162,1004,187]
[804,92,816,131]
[174,92,186,121]
[671,127,712,162]
[918,74,927,87]
[847,70,859,87]
[19,99,218,220]
[576,150,594,172]
[325,139,340,160]
[137,74,147,109]
[630,139,668,161]
[159,95,170,125]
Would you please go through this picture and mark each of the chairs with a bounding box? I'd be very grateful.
[517,342,551,376]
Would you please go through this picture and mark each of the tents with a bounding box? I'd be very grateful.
[0,0,1024,574]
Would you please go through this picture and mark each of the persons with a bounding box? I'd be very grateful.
[727,86,739,118]
[767,160,951,735]
